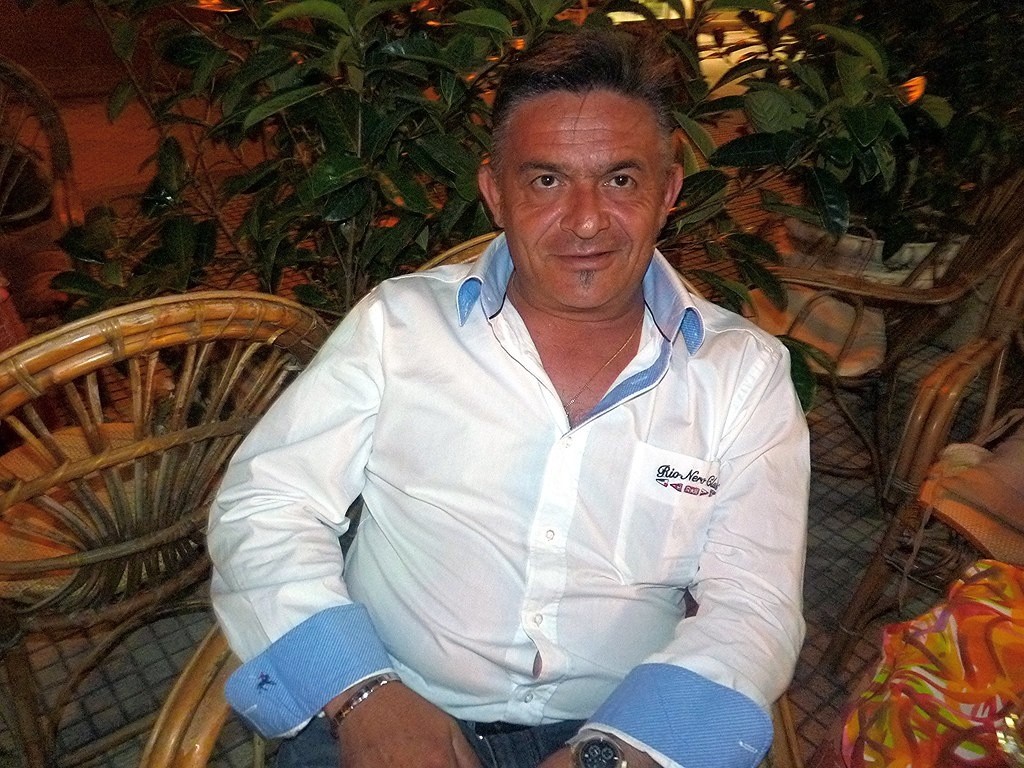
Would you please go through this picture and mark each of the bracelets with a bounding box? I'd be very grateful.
[330,672,402,741]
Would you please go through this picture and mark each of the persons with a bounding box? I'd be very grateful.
[208,25,810,768]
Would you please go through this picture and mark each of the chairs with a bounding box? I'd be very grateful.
[0,290,331,768]
[134,231,804,768]
[740,163,1024,690]
[0,52,87,238]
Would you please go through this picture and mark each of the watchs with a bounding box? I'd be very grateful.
[564,729,628,768]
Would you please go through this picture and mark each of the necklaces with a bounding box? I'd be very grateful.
[564,313,646,420]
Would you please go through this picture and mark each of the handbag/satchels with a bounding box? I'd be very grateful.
[841,558,1024,767]
